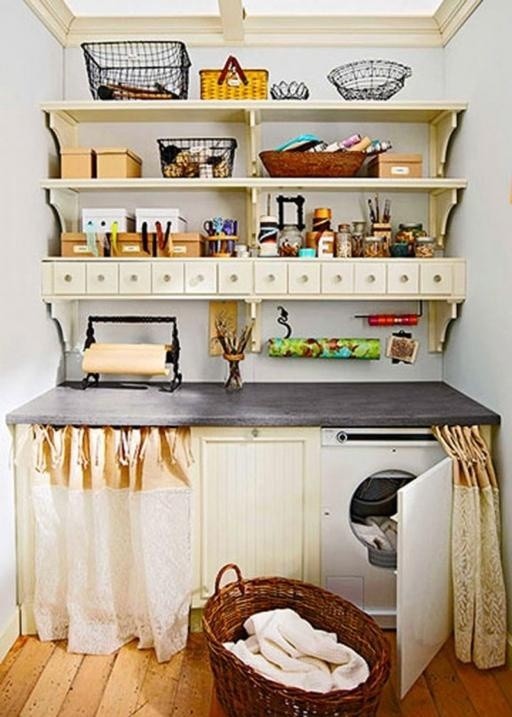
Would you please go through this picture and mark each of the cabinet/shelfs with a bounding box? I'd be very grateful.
[188,425,323,614]
[4,380,502,700]
[0,100,469,353]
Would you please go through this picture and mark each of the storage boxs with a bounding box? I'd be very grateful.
[157,139,238,178]
[80,41,192,100]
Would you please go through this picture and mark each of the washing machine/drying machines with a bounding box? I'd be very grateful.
[326,429,449,629]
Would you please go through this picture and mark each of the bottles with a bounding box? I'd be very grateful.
[260,208,438,259]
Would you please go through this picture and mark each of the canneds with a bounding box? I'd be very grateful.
[363,237,384,258]
[394,223,428,256]
[413,236,435,257]
